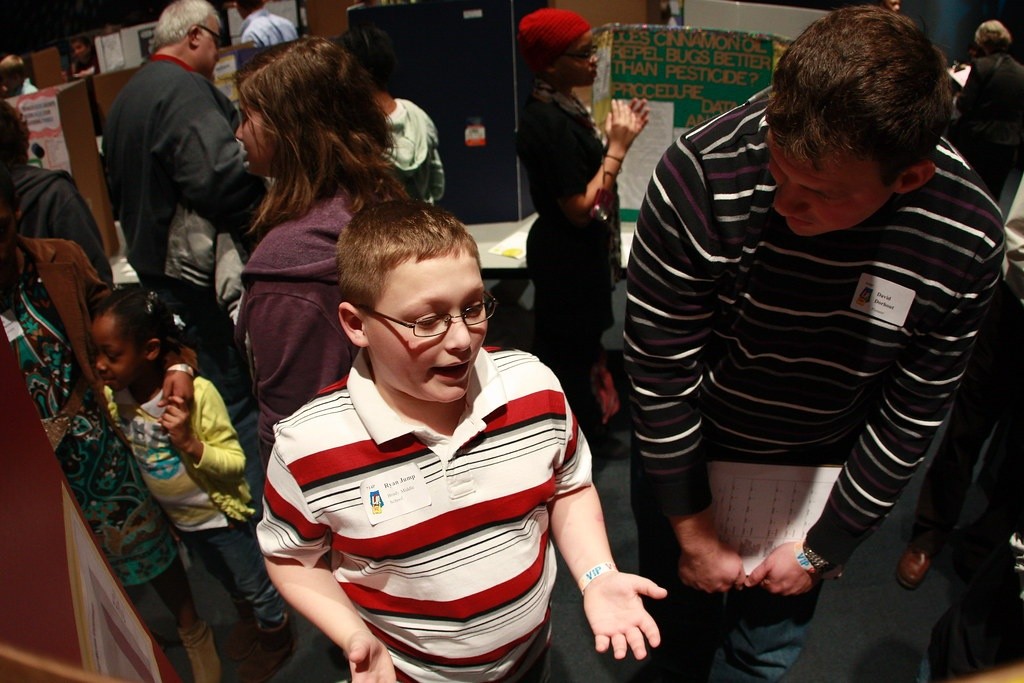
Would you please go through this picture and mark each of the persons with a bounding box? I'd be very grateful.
[256,196,668,683]
[622,5,1009,557]
[516,8,649,458]
[0,0,445,683]
[885,0,1024,683]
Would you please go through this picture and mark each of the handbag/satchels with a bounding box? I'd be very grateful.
[948,111,993,159]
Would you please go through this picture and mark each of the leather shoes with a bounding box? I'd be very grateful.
[895,531,941,589]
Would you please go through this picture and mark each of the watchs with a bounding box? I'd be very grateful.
[802,539,837,575]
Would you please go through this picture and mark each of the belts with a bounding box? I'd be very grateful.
[36,377,87,455]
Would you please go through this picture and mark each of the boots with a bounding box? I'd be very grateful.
[223,620,260,660]
[237,612,298,683]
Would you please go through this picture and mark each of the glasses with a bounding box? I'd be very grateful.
[197,25,223,48]
[561,45,598,59]
[362,289,497,338]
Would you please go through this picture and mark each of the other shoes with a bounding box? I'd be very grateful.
[178,616,222,683]
[589,436,630,459]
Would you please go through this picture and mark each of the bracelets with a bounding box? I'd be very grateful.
[578,562,618,596]
[166,363,195,377]
[794,541,844,580]
[603,153,623,163]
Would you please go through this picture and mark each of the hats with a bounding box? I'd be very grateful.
[515,8,594,73]
